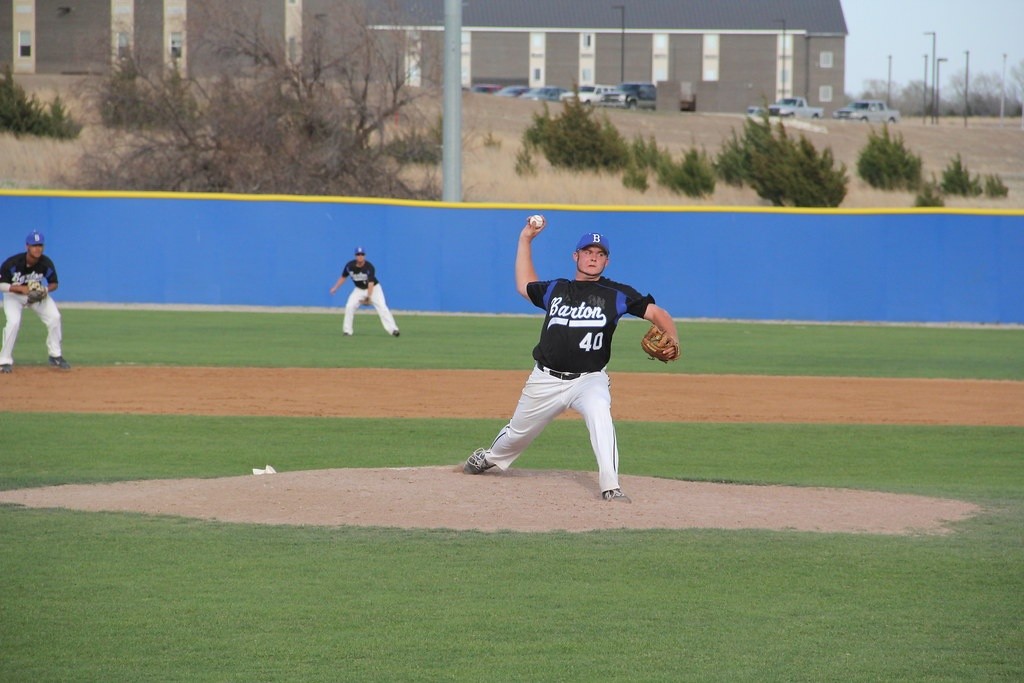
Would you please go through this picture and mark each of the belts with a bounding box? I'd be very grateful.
[537,362,581,381]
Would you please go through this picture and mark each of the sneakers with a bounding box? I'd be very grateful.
[462,448,497,475]
[602,489,632,504]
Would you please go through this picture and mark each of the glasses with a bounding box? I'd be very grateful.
[355,253,365,256]
[30,244,43,246]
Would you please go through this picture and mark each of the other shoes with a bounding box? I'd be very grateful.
[343,333,348,336]
[49,357,70,369]
[393,329,400,336]
[0,365,11,374]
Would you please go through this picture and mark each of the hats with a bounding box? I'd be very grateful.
[575,232,611,256]
[25,231,44,244]
[354,245,366,253]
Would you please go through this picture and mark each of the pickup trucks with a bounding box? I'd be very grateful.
[833,99,900,125]
[766,97,824,121]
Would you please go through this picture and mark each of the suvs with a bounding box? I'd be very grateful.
[600,83,657,110]
[559,83,616,105]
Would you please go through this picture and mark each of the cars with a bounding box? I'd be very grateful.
[519,87,567,101]
[494,86,529,97]
[473,84,504,93]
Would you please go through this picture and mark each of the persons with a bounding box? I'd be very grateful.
[462,213,681,505]
[0,232,70,373]
[331,247,400,337]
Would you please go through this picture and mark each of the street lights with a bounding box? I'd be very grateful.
[935,57,949,125]
[923,32,936,124]
[611,5,625,84]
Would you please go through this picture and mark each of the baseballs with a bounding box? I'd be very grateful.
[529,215,543,229]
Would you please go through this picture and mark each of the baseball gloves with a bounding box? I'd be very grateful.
[25,278,47,304]
[359,297,371,306]
[641,326,681,363]
